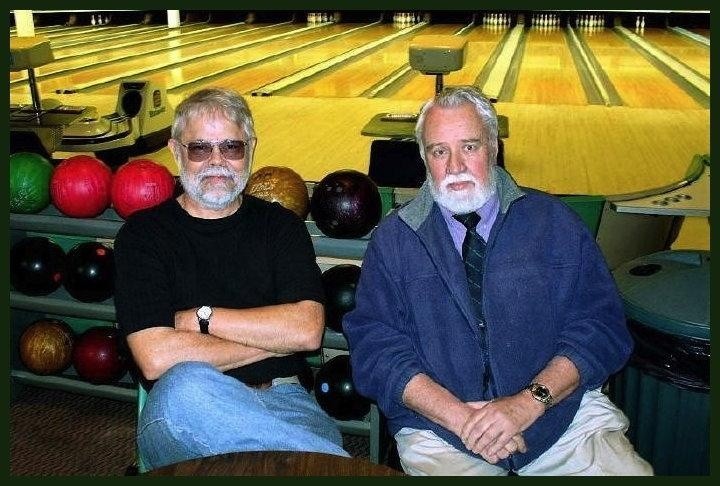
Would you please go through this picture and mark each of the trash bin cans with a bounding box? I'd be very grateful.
[612,249,712,477]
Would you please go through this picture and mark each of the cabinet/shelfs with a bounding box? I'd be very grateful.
[11,205,394,465]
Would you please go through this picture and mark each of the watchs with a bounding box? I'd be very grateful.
[195,303,213,335]
[526,381,557,408]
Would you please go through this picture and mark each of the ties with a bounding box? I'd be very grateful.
[451,211,487,333]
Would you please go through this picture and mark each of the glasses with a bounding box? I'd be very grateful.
[177,137,255,162]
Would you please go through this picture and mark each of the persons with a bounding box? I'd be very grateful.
[113,86,352,472]
[343,80,655,477]
